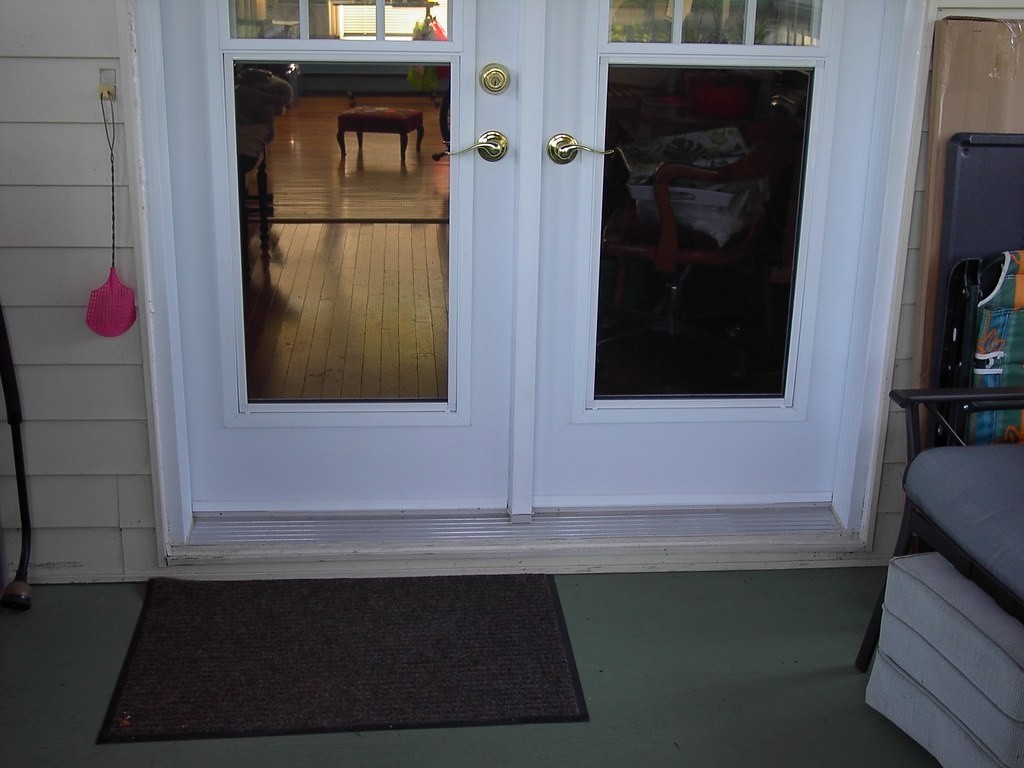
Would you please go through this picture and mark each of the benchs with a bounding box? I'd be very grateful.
[236,68,292,259]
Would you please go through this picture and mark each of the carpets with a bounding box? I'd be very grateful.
[96,574,589,750]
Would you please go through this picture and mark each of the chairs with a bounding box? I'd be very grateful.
[602,115,806,312]
[853,384,1024,768]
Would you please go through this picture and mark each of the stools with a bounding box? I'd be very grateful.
[335,106,425,160]
